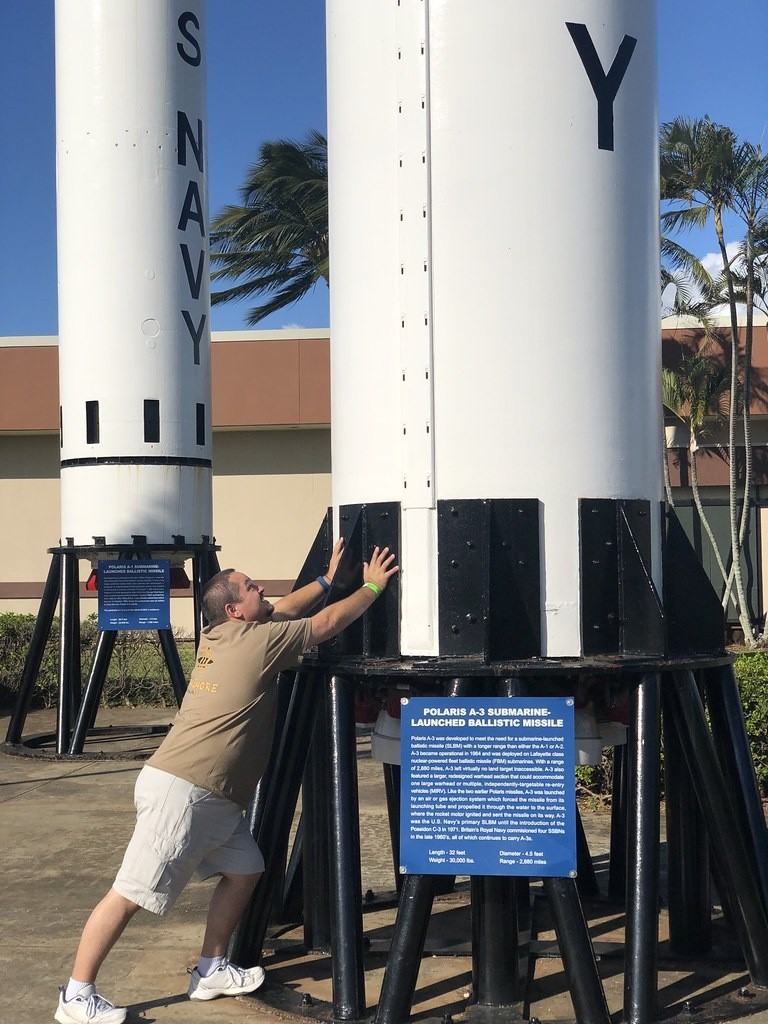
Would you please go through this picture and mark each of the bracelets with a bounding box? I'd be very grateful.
[317,576,329,592]
[362,582,382,598]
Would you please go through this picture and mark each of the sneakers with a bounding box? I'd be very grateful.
[185,956,267,1002]
[52,982,128,1024]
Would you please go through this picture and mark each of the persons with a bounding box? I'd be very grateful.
[50,534,399,1022]
